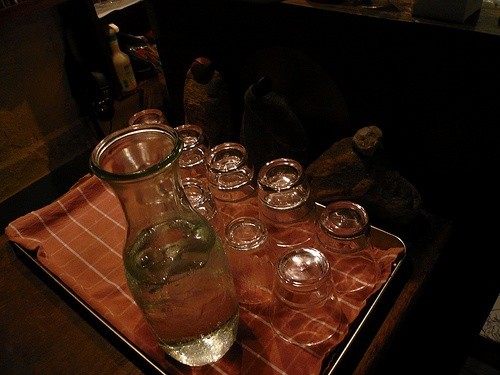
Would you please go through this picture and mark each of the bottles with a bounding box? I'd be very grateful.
[90,124,240,366]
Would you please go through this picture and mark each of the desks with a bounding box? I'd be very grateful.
[0,150,450,375]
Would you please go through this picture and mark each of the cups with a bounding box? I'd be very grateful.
[127,108,171,203]
[205,141,257,216]
[178,184,222,242]
[259,159,317,244]
[220,217,276,304]
[271,247,342,345]
[311,200,378,296]
[166,125,208,181]
